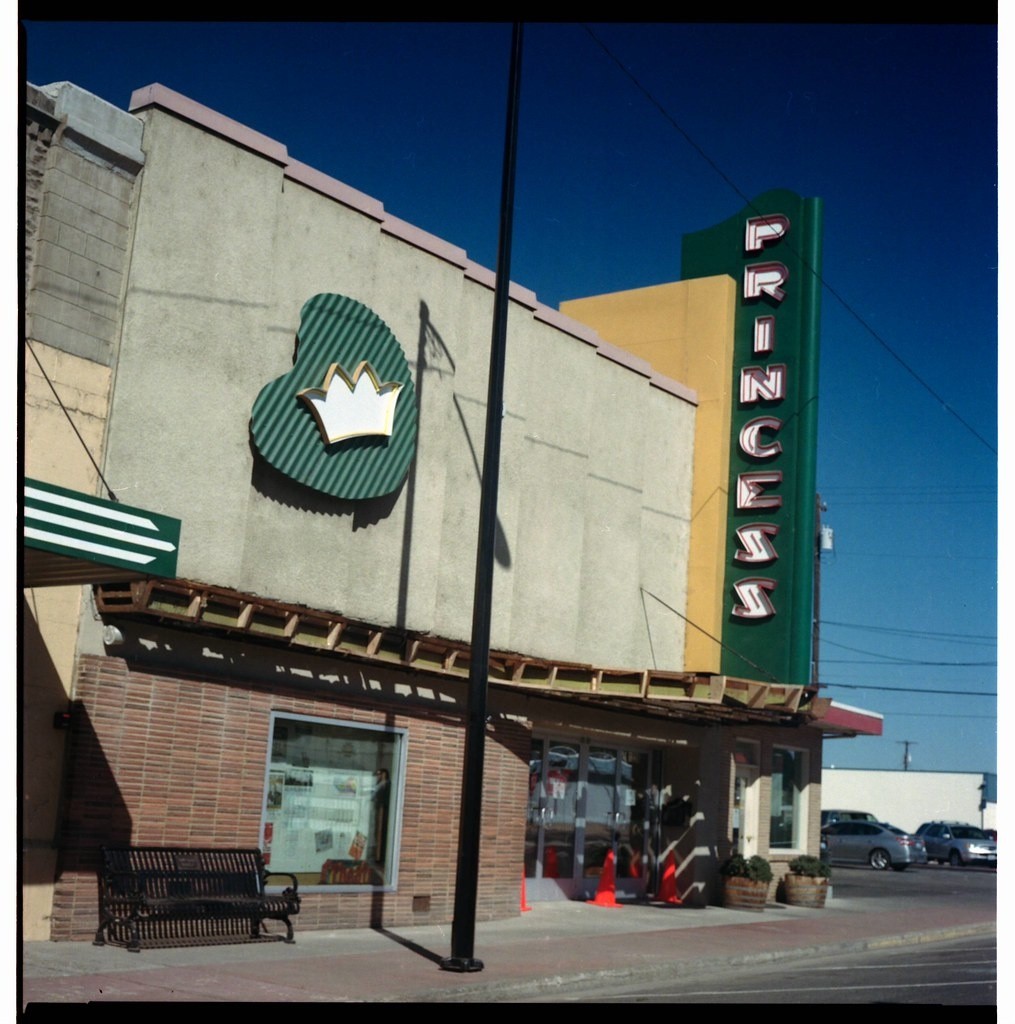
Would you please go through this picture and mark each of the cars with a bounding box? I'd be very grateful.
[819,821,928,871]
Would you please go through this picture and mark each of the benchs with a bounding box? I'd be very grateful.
[93,843,302,952]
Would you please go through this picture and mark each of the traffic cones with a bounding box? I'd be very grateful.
[650,849,683,904]
[546,847,558,878]
[520,869,532,911]
[586,850,623,908]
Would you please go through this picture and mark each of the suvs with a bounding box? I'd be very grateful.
[822,810,880,841]
[917,818,1000,867]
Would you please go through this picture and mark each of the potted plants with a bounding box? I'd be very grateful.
[720,854,774,910]
[785,855,830,909]
